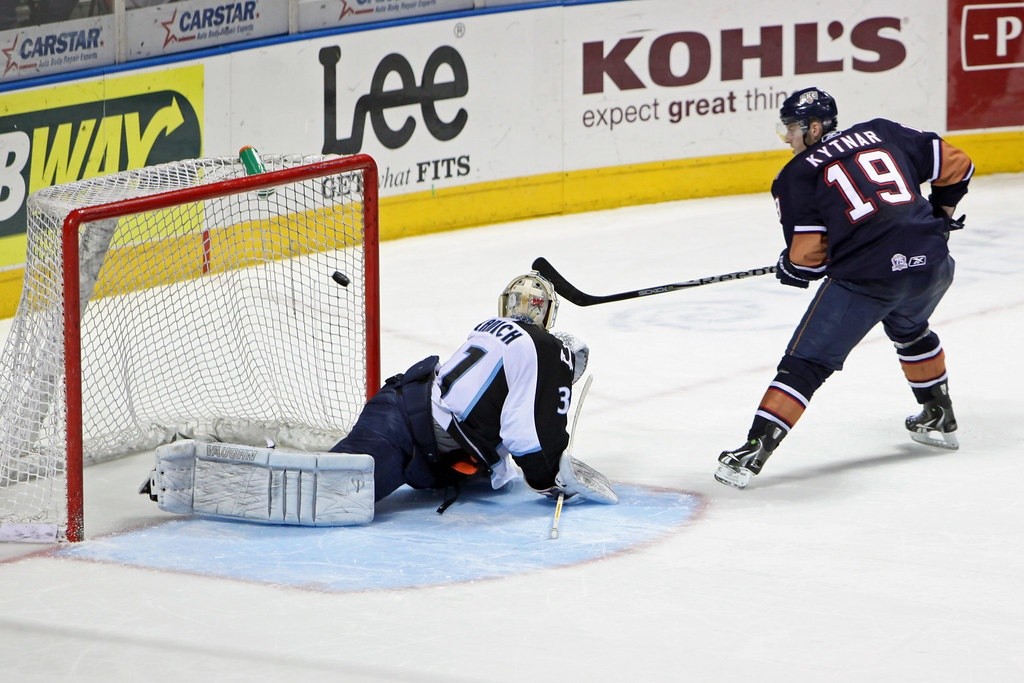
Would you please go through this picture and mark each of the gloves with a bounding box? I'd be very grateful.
[523,449,619,505]
[554,331,589,384]
[776,248,826,289]
[933,205,965,230]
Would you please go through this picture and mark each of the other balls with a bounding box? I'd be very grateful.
[332,270,351,288]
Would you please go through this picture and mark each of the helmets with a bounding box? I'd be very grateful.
[780,87,838,148]
[498,270,559,329]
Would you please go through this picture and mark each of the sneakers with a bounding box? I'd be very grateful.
[714,421,787,489]
[905,398,960,450]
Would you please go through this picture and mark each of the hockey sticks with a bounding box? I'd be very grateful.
[533,214,964,307]
[549,373,595,540]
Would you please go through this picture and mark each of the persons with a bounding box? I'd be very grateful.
[329,270,620,512]
[717,84,975,490]
